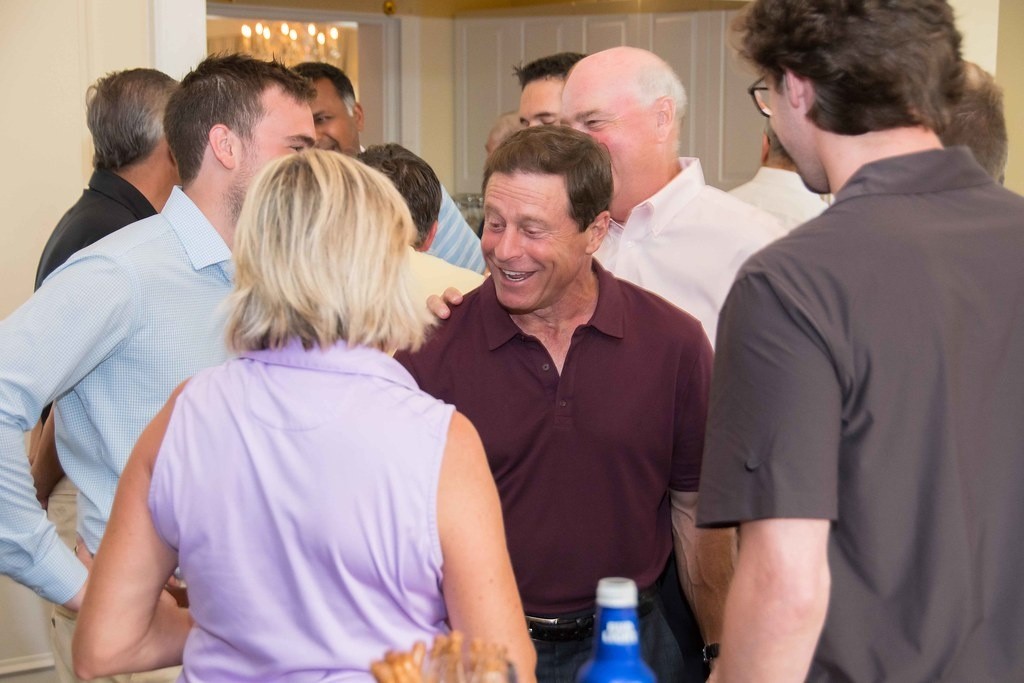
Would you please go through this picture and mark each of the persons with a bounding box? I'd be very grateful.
[397,124,740,683]
[511,52,586,127]
[0,54,317,682]
[931,58,1009,186]
[33,68,182,296]
[355,143,491,305]
[426,46,783,351]
[73,147,537,683]
[485,112,523,153]
[288,62,492,279]
[726,113,831,224]
[690,1,1024,683]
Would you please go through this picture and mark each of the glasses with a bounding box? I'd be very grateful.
[746,72,771,118]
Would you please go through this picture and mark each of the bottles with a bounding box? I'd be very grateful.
[574,576,661,683]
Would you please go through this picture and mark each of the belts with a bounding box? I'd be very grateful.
[521,600,652,642]
[163,585,189,608]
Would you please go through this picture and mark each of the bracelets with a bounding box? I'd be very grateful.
[701,643,721,663]
